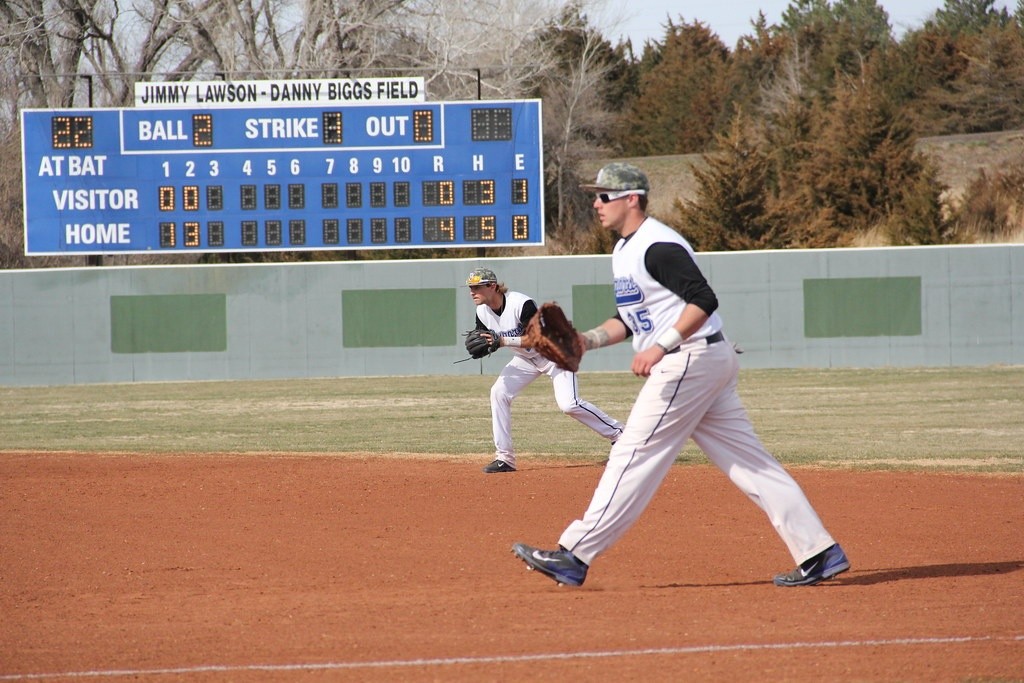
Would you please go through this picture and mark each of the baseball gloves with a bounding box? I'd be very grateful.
[526,302,583,374]
[465,329,501,359]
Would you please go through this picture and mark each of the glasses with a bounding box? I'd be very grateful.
[595,189,645,203]
[466,276,496,283]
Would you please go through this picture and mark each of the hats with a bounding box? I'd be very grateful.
[459,268,497,287]
[578,163,649,192]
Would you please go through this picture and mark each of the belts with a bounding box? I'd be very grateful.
[664,331,724,354]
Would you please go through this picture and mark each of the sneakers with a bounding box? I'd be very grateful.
[510,542,589,587]
[774,543,850,587]
[483,460,516,473]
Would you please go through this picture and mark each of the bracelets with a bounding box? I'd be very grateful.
[504,337,521,348]
[657,327,684,352]
[581,326,609,351]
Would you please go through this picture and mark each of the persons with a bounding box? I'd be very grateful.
[510,163,853,588]
[466,267,625,473]
